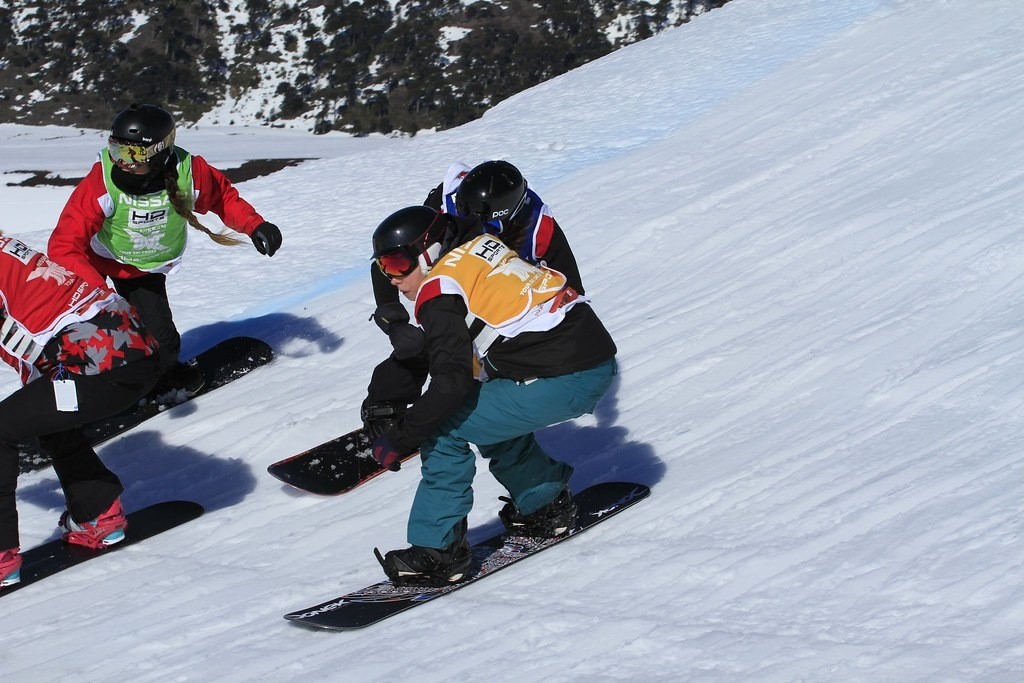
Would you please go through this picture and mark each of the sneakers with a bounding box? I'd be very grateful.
[58,511,126,545]
[362,394,416,456]
[498,491,578,537]
[1,549,23,587]
[384,547,472,589]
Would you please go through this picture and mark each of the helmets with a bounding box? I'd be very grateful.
[369,205,452,278]
[108,102,175,176]
[455,160,529,235]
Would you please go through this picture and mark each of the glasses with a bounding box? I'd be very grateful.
[109,142,147,173]
[375,249,419,281]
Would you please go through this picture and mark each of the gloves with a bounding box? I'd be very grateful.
[251,221,283,257]
[390,325,427,362]
[370,436,401,472]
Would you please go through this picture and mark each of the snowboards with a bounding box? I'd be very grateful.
[0,497,207,599]
[265,407,423,497]
[15,334,277,477]
[281,477,651,634]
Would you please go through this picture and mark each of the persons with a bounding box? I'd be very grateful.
[361,160,585,437]
[372,205,619,574]
[0,230,161,587]
[47,104,283,397]
